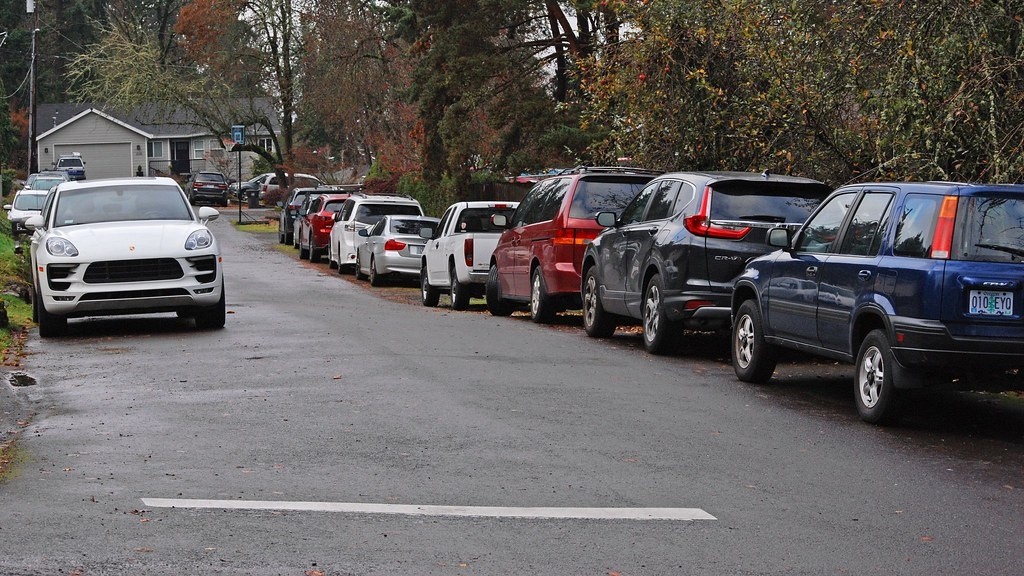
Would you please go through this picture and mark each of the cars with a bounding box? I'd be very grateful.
[354,214,443,287]
[419,201,523,309]
[290,191,352,265]
[227,173,271,201]
[3,152,86,237]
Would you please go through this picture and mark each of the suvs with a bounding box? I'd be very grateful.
[327,194,425,274]
[483,166,673,325]
[279,187,345,245]
[259,173,328,202]
[185,170,232,206]
[22,176,226,337]
[579,168,861,355]
[727,179,1024,428]
